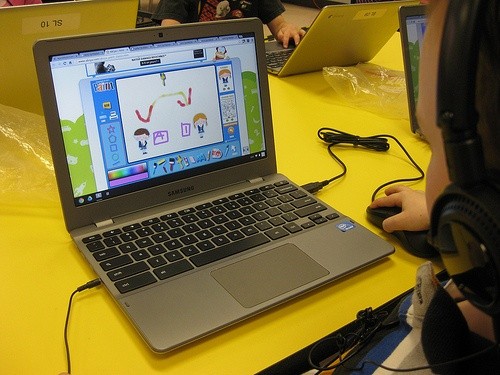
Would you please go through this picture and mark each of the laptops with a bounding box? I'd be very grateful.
[399,4,430,140]
[266,0,420,77]
[0,0,139,118]
[32,17,396,355]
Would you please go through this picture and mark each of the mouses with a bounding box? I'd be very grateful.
[366,206,435,258]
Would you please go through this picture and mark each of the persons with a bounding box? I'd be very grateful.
[151,0,308,48]
[369,1,500,375]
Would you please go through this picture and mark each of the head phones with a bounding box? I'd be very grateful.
[431,0,500,315]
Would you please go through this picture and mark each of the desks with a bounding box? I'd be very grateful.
[0,31,446,375]
